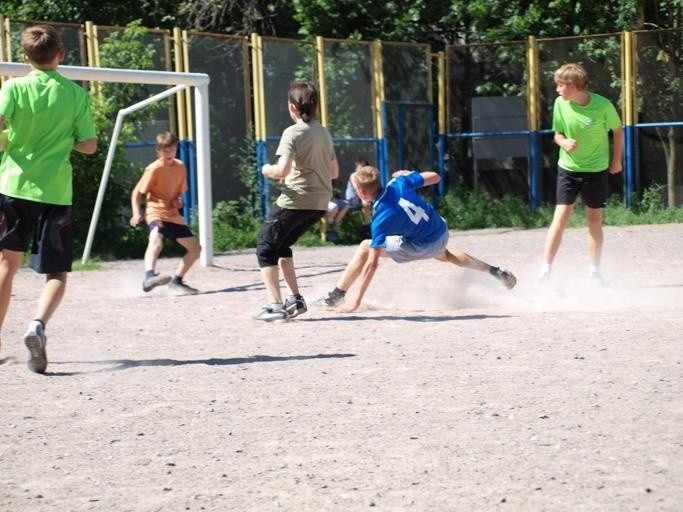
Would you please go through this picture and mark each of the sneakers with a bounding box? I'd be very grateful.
[246,303,290,324]
[279,295,308,318]
[167,282,200,295]
[499,269,517,290]
[23,322,48,374]
[142,273,170,292]
[311,291,345,311]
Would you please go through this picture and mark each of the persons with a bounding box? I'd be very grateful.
[344,157,373,241]
[252,81,340,322]
[535,62,625,287]
[0,23,98,373]
[309,165,517,314]
[322,194,351,244]
[127,132,200,294]
